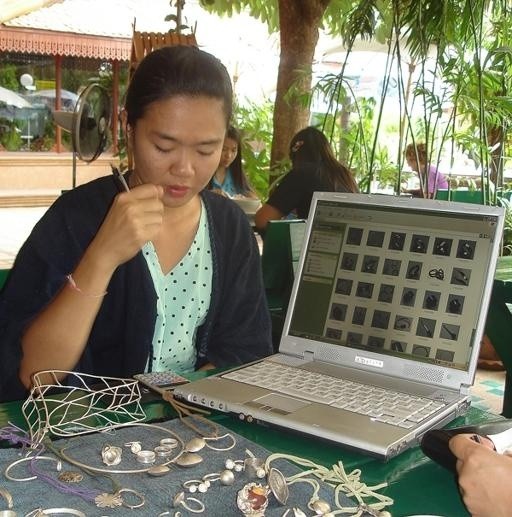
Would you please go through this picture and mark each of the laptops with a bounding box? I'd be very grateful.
[174,191,507,464]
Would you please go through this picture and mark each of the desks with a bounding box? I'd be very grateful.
[0,364,506,514]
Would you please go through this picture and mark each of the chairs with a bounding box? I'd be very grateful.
[257,217,304,314]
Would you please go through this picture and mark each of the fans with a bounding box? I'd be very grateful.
[48,81,112,192]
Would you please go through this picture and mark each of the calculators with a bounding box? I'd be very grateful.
[131,370,191,398]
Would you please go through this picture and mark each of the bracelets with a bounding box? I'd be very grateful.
[67,273,107,299]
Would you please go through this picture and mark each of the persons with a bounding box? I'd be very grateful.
[255,126,360,228]
[450,434,511,516]
[0,46,274,402]
[402,144,448,198]
[204,126,256,199]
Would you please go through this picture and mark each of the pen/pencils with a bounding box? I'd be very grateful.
[109,162,130,191]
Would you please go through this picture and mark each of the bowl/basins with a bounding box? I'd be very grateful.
[230,197,262,215]
